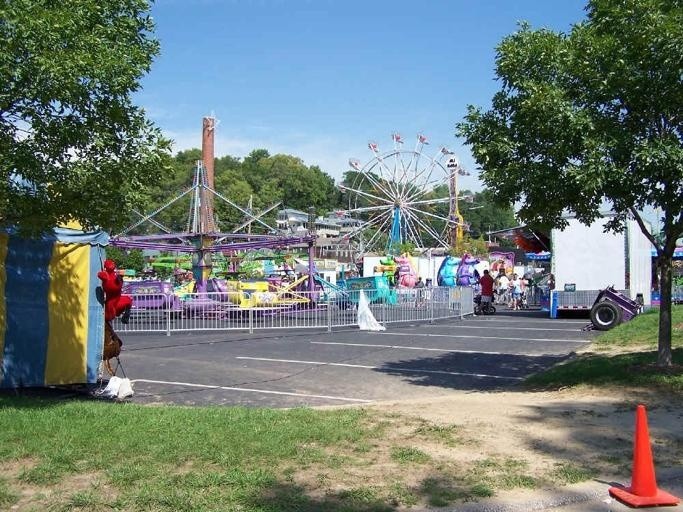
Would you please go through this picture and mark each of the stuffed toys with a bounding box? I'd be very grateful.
[97,259,130,324]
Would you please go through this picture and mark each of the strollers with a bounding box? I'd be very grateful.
[472,294,496,315]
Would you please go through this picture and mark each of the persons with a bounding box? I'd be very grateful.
[384,267,533,314]
